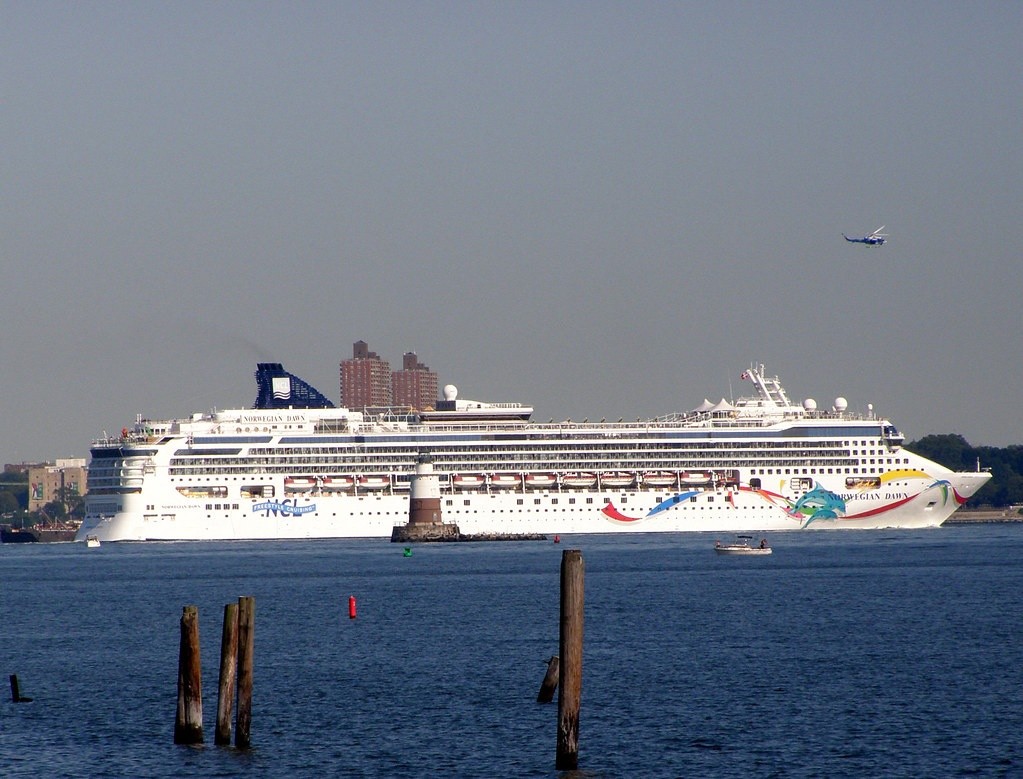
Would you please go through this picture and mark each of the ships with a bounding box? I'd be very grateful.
[73,359,994,544]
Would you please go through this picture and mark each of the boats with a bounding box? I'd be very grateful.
[85,534,102,548]
[284,475,317,489]
[357,475,390,490]
[716,473,737,487]
[320,475,354,490]
[680,469,713,486]
[599,471,635,487]
[559,472,596,487]
[452,474,485,489]
[524,473,556,489]
[488,474,522,489]
[714,543,773,555]
[641,470,677,486]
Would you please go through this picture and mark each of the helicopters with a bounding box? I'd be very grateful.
[841,223,890,248]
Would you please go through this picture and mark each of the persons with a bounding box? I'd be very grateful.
[760,539,767,549]
